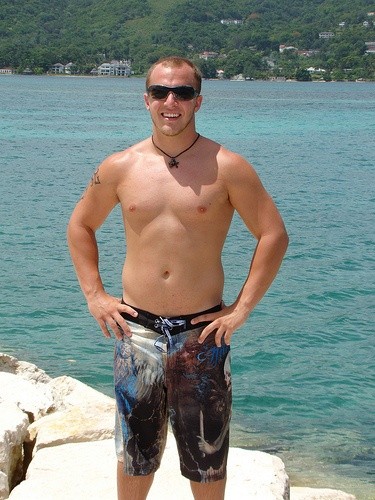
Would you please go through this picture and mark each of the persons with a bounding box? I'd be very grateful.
[65,55,289,500]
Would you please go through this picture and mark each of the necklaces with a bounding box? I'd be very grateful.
[151,133,200,168]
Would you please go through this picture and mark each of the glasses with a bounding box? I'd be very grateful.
[146,85,200,102]
